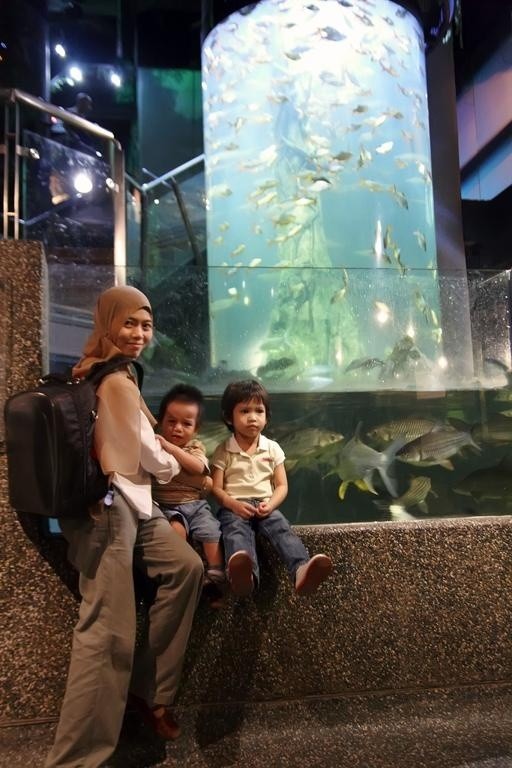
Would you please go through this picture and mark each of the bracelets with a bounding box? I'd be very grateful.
[203,476,207,491]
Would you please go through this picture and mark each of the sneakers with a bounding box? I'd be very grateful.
[228,551,254,594]
[295,554,332,594]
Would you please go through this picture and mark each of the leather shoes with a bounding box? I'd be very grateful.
[140,700,181,740]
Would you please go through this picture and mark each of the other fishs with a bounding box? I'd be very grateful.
[200,0,443,382]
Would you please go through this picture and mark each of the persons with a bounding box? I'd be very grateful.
[43,285,214,767]
[213,379,332,597]
[153,384,225,593]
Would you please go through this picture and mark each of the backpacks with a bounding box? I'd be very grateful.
[4,357,143,517]
